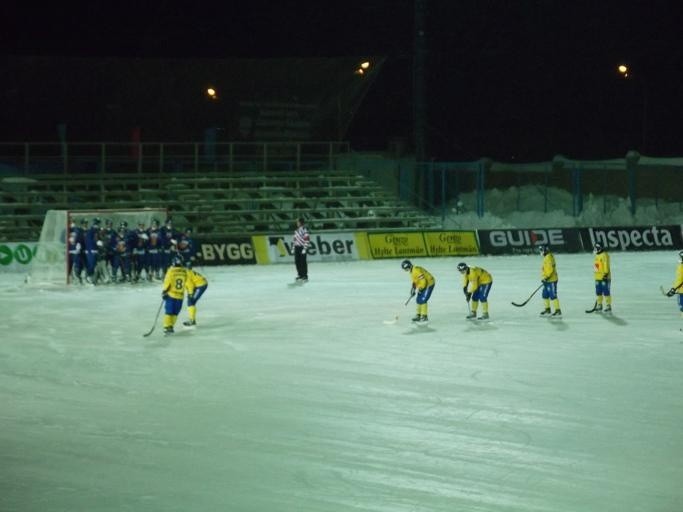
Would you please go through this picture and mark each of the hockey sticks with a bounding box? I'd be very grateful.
[463,301,473,331]
[660,285,682,295]
[383,295,414,325]
[511,283,545,306]
[586,300,597,313]
[143,298,165,337]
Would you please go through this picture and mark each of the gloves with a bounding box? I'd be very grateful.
[412,288,415,296]
[162,290,168,298]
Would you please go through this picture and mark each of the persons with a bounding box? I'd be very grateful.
[666,250,682,332]
[290,217,310,280]
[161,255,187,336]
[538,245,561,317]
[401,259,435,321]
[592,241,612,312]
[164,264,208,326]
[457,262,492,320]
[69,217,197,286]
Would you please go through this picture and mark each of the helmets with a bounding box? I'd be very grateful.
[593,243,603,254]
[539,245,549,256]
[457,263,466,271]
[402,261,411,268]
[69,216,193,234]
[172,257,182,266]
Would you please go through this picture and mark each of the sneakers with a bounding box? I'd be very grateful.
[163,320,196,333]
[413,305,613,322]
